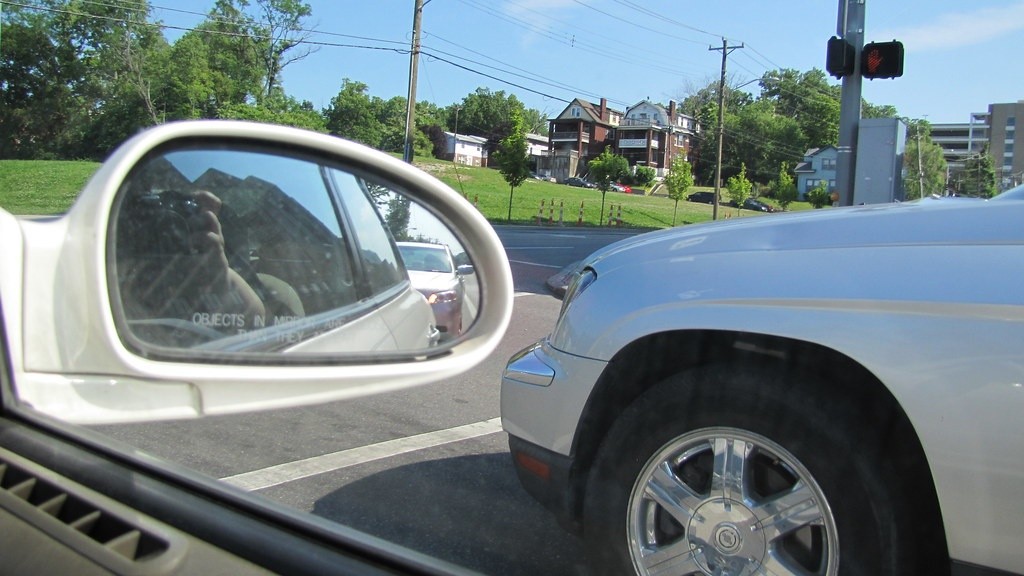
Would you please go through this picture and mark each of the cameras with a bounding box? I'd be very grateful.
[126,190,205,255]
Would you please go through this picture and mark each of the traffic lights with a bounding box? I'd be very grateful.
[864,42,899,79]
[825,35,846,77]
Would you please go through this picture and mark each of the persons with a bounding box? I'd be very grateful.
[119,166,270,348]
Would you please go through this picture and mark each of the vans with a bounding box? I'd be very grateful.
[686,191,722,205]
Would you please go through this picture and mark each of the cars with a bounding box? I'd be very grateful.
[396,239,478,343]
[729,197,774,213]
[527,171,632,193]
[116,149,439,357]
[501,178,1024,575]
